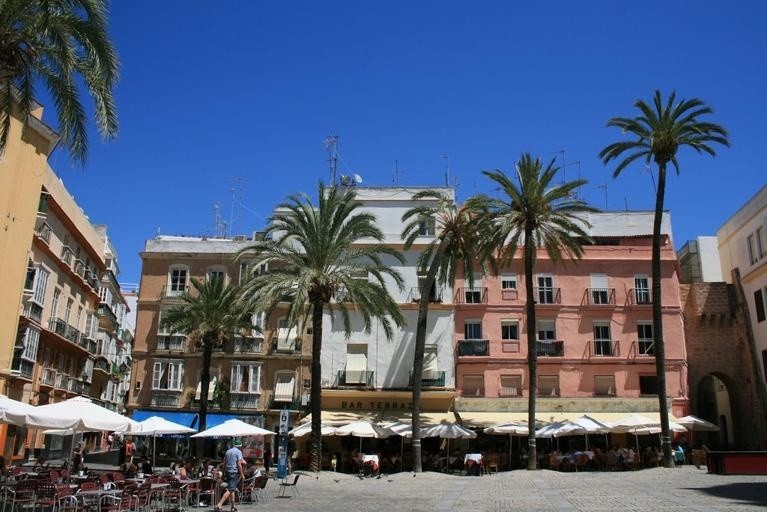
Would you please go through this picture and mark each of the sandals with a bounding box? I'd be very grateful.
[213,505,222,511]
[231,507,237,512]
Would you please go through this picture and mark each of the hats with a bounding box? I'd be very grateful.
[233,440,243,447]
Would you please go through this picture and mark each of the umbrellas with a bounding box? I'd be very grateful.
[0,395,277,485]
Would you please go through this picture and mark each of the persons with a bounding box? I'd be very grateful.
[214,441,245,512]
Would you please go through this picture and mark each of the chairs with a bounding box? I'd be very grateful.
[277,474,300,498]
[0,467,272,512]
